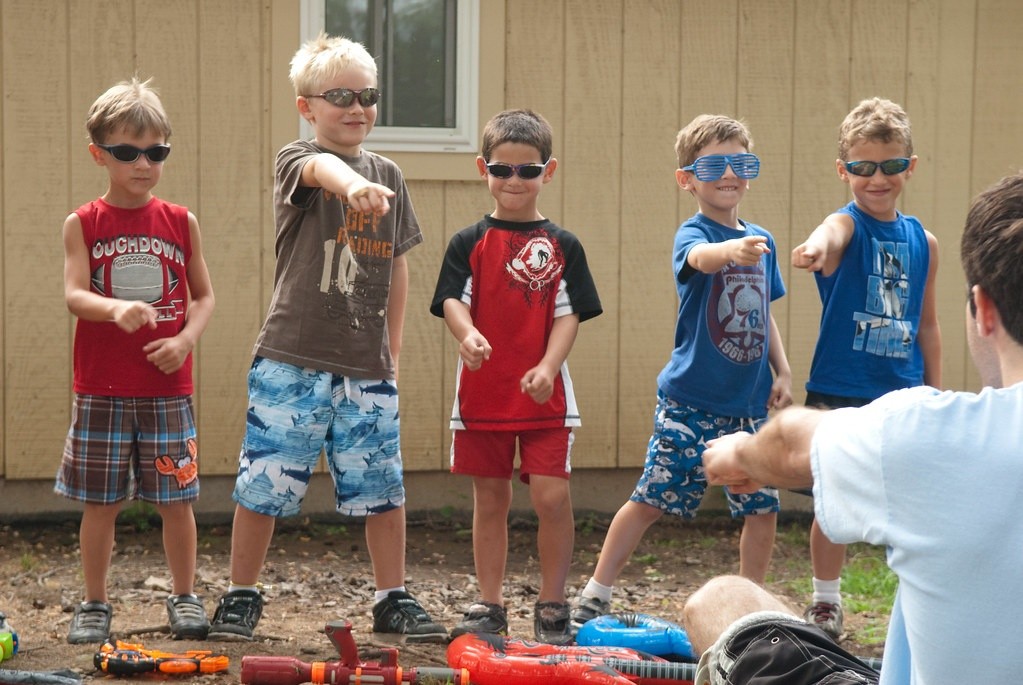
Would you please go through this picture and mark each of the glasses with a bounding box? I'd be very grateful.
[485,161,547,179]
[97,143,171,164]
[683,153,760,181]
[845,158,910,176]
[306,88,381,108]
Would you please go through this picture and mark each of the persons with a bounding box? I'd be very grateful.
[571,113,792,639]
[682,169,1023,685]
[429,108,605,640]
[208,38,448,642]
[791,97,943,641]
[52,84,209,644]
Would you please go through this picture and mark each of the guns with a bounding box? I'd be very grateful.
[239,620,471,685]
[91,633,230,675]
[1,608,19,663]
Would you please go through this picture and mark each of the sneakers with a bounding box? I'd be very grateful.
[803,601,847,644]
[533,600,574,645]
[373,589,448,642]
[67,601,113,643]
[165,594,210,640]
[210,590,264,642]
[451,601,509,653]
[570,596,611,639]
